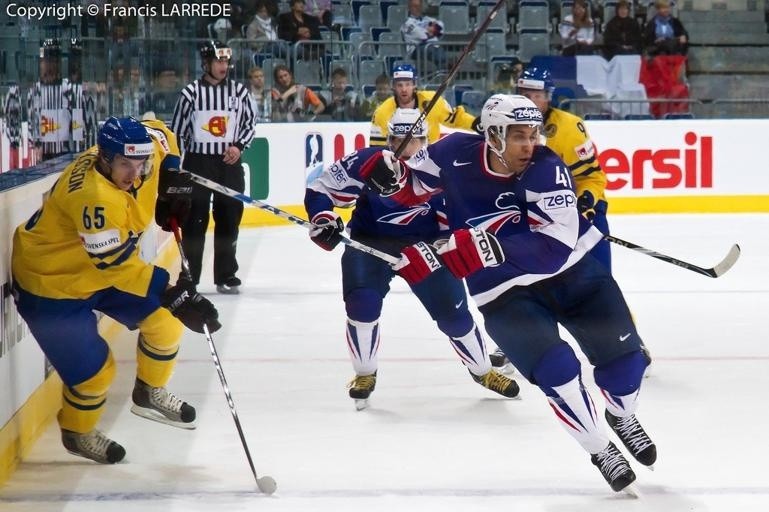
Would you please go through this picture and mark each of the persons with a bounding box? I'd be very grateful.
[10,116,222,463]
[302,109,523,398]
[371,60,484,167]
[0,36,196,179]
[357,91,659,494]
[171,38,257,292]
[487,65,613,366]
[197,0,694,121]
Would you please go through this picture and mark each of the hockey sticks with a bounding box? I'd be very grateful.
[179,134,400,269]
[169,216,277,495]
[603,235,741,278]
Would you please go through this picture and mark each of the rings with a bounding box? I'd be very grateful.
[227,150,235,158]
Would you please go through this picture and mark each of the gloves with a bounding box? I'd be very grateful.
[360,150,409,198]
[434,228,505,278]
[162,282,221,334]
[390,240,441,285]
[308,211,344,253]
[155,172,193,232]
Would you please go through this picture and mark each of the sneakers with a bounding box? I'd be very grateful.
[176,270,192,283]
[471,371,520,397]
[132,376,196,422]
[214,274,241,287]
[62,427,126,463]
[604,408,656,466]
[347,374,376,398]
[489,347,510,367]
[589,440,636,492]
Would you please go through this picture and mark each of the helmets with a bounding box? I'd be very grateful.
[475,93,544,155]
[391,63,418,91]
[516,68,556,104]
[97,117,157,178]
[387,107,428,158]
[200,39,233,65]
